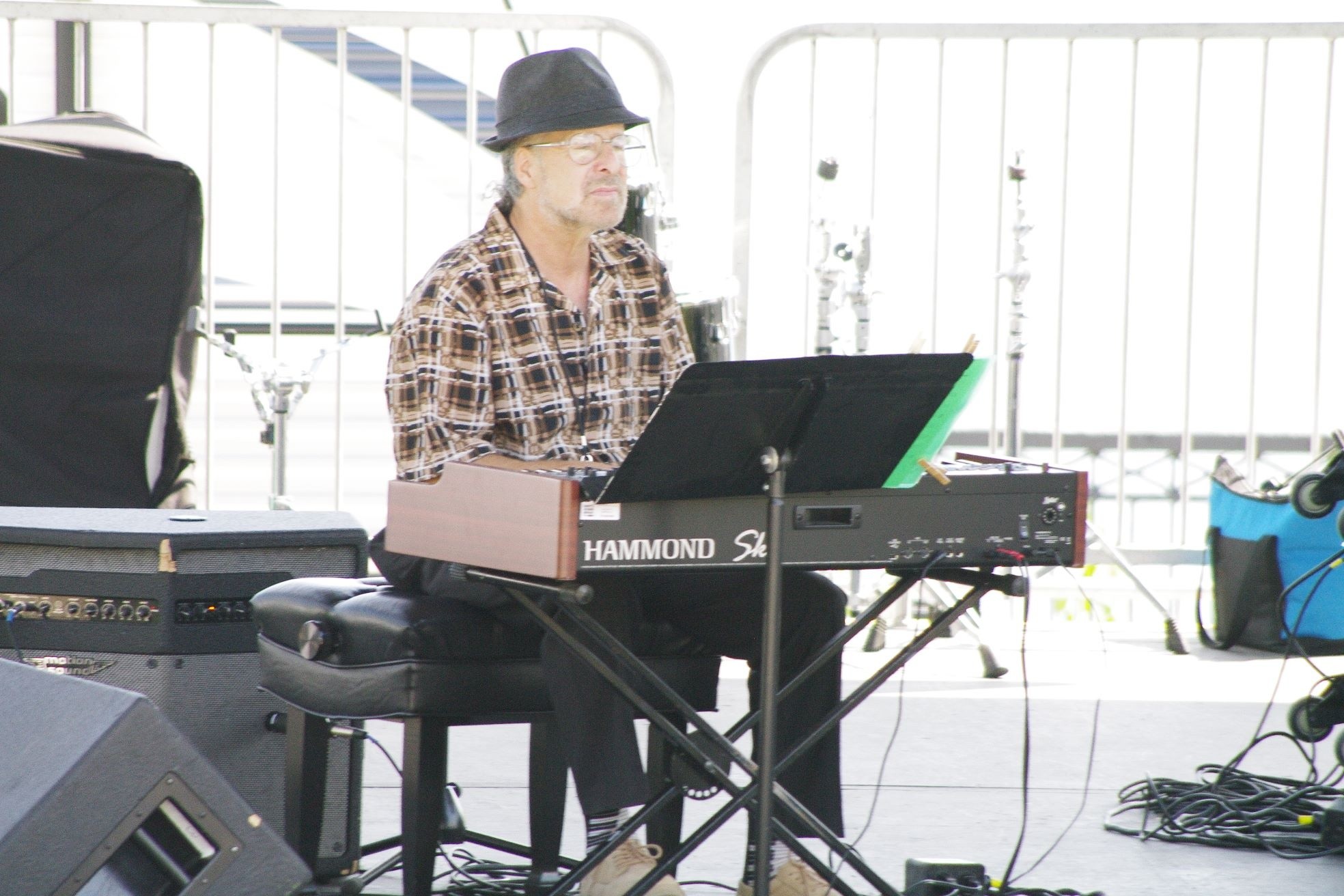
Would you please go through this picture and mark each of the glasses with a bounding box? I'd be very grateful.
[522,132,646,166]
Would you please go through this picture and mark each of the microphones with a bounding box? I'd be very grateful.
[265,712,368,741]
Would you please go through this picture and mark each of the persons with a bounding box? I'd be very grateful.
[382,48,849,896]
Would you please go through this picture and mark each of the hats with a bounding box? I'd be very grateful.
[479,47,650,153]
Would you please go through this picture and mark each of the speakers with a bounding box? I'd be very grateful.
[0,511,368,896]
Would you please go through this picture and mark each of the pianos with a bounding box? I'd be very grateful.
[379,351,1092,896]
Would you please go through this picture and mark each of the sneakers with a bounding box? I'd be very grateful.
[580,837,685,896]
[736,860,842,896]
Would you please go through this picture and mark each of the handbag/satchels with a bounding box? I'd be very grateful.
[1196,435,1343,658]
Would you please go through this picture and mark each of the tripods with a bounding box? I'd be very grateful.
[339,784,582,896]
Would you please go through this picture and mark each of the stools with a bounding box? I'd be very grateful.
[248,578,723,896]
